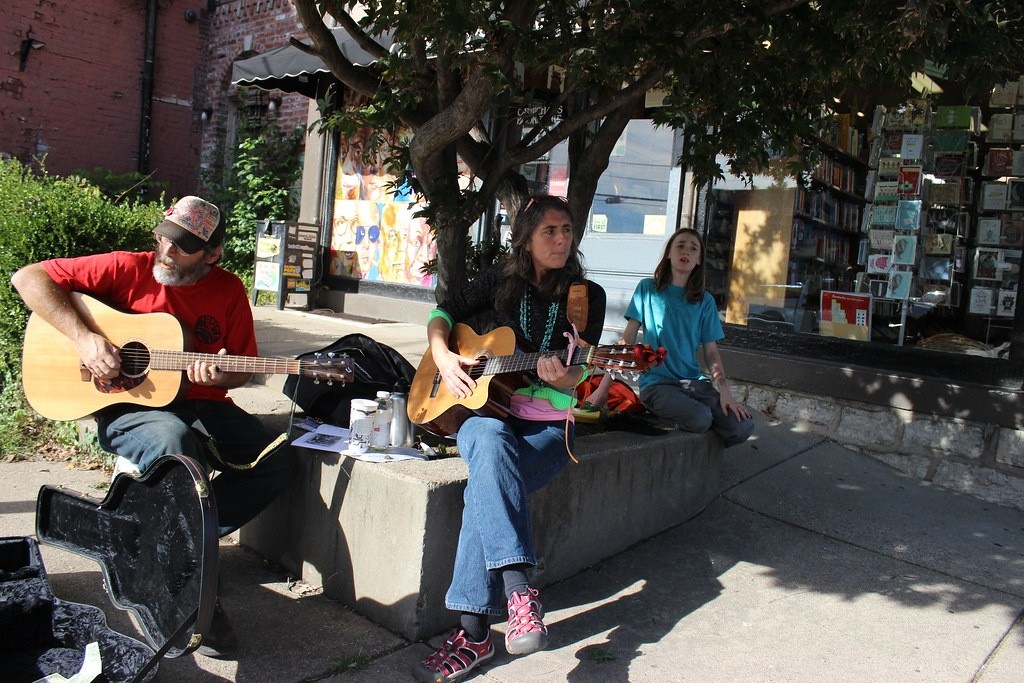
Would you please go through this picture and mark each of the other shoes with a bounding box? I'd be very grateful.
[194,596,239,656]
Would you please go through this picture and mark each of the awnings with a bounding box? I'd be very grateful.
[232,0,611,95]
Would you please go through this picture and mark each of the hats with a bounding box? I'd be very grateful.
[151,194,226,254]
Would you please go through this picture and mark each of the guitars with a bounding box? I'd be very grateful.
[19,289,359,427]
[406,320,668,439]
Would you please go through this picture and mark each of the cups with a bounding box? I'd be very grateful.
[348,399,379,454]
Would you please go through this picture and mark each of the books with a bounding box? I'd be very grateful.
[786,74,1024,317]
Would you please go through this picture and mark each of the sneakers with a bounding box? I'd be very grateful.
[411,628,495,683]
[504,586,550,654]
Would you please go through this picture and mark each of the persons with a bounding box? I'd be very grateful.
[585,228,755,445]
[411,194,607,683]
[10,195,282,655]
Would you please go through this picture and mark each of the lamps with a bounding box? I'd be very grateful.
[268,96,282,116]
[200,106,213,122]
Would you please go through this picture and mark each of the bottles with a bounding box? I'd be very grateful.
[390,386,406,447]
[372,391,393,449]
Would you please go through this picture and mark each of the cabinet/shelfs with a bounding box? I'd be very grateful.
[723,75,1023,361]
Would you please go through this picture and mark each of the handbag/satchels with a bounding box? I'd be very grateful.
[283,335,416,429]
